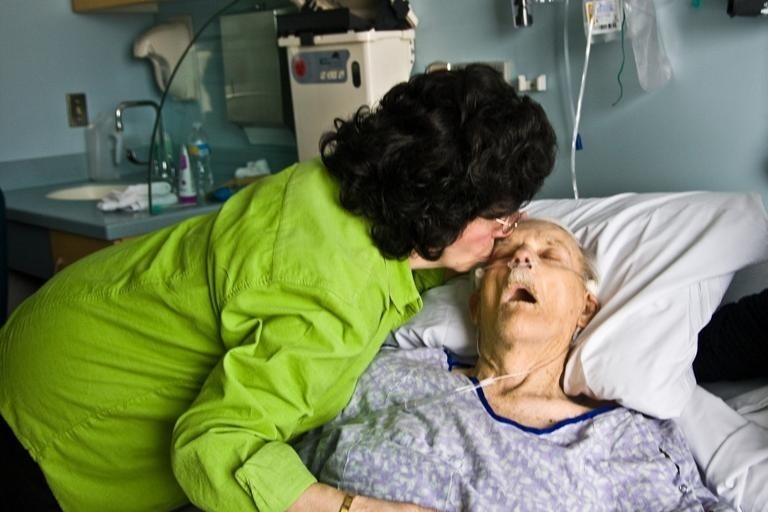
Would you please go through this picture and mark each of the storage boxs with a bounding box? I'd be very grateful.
[277,4,419,162]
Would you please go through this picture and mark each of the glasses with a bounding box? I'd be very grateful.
[494,215,520,234]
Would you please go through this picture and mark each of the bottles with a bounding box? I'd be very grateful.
[189,119,215,205]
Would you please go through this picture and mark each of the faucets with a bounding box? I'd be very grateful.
[114,98,176,181]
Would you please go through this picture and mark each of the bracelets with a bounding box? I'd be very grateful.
[340,491,356,512]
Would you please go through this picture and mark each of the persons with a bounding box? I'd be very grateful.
[289,217,741,512]
[0,61,559,512]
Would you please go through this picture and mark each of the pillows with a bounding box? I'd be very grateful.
[377,190,768,419]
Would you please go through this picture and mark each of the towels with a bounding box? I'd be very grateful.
[97,181,178,212]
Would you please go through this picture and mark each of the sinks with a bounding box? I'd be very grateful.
[43,178,134,202]
